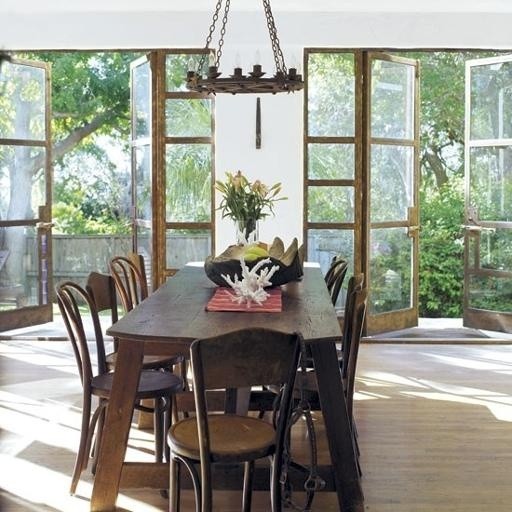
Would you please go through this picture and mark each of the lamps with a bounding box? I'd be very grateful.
[185,0,307,96]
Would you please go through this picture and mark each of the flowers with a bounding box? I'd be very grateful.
[212,168,289,245]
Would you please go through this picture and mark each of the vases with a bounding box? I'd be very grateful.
[232,219,261,244]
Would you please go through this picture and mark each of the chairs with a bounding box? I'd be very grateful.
[54,276,182,497]
[293,272,365,438]
[108,255,148,314]
[255,286,372,477]
[86,271,184,465]
[163,325,303,512]
[324,258,349,306]
